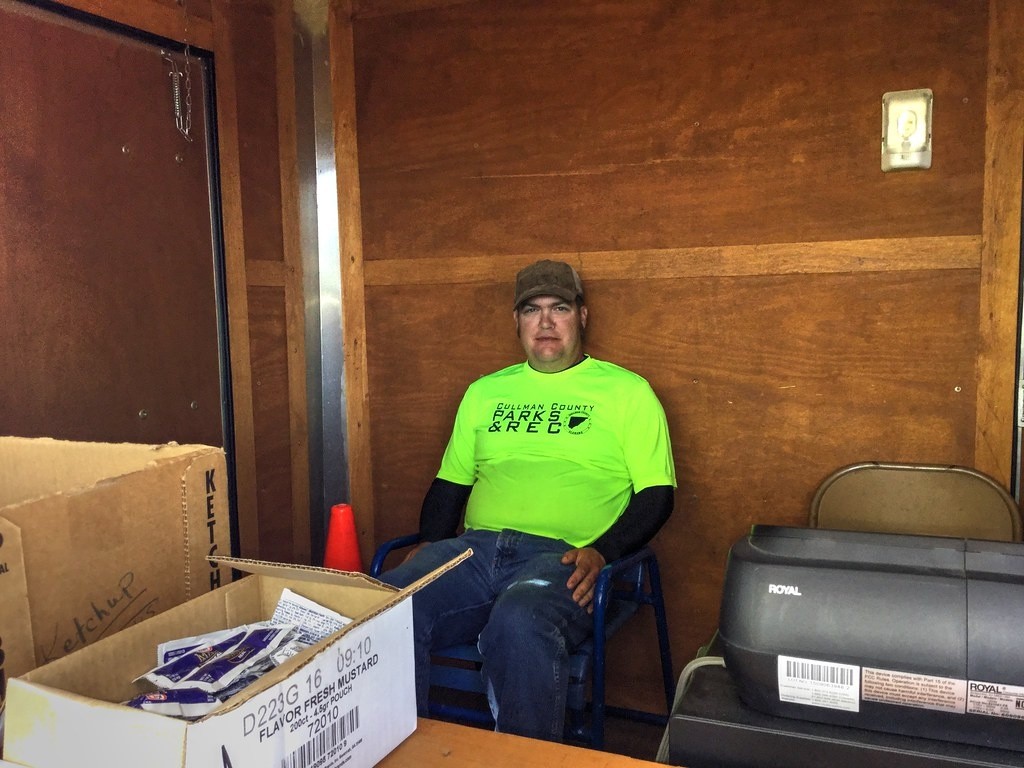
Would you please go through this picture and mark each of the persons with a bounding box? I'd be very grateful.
[376,259,678,743]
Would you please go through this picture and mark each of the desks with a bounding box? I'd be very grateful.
[372,715,683,768]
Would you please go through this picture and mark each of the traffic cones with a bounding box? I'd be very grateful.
[324,501,362,574]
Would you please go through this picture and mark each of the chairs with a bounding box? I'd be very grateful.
[808,462,1024,544]
[370,530,678,755]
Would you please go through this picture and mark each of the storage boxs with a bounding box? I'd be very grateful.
[0,546,477,768]
[1,434,233,762]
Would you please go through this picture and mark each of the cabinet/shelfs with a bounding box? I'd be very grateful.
[666,628,1024,768]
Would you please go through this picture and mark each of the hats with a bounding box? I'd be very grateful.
[513,258,586,310]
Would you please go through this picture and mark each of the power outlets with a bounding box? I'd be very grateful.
[881,88,936,172]
